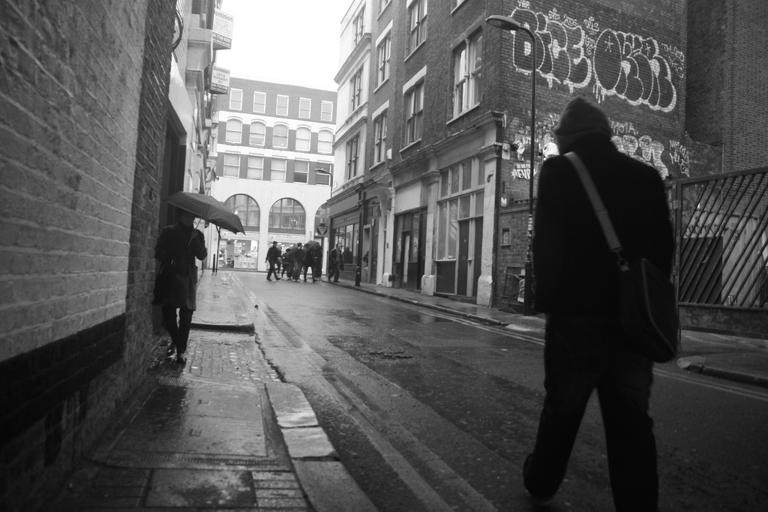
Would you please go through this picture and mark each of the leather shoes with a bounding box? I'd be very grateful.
[167,341,176,356]
[177,352,187,364]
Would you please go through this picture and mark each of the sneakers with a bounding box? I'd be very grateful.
[524,452,554,501]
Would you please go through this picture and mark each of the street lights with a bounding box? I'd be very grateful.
[314,169,335,283]
[484,15,537,317]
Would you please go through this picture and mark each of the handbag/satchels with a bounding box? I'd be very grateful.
[619,254,681,363]
[151,270,189,306]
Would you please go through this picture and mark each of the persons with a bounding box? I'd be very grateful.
[262,238,345,284]
[152,209,208,367]
[519,96,676,512]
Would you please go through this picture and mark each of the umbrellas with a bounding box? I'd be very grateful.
[166,190,247,237]
